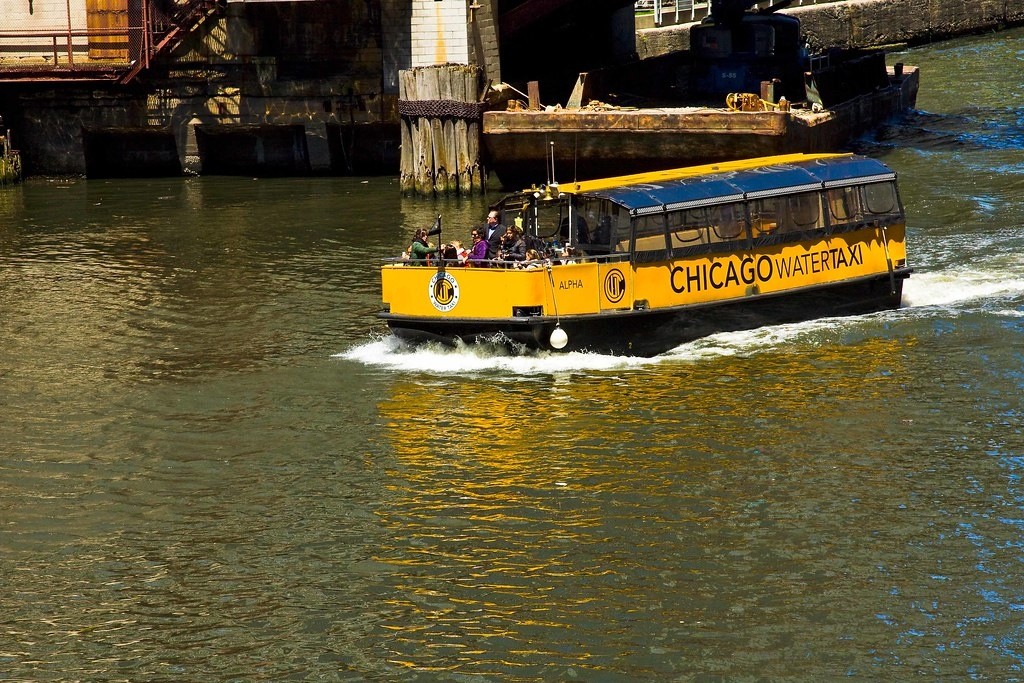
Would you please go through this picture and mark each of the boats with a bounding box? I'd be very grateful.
[374,151,913,353]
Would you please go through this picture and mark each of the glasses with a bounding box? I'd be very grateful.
[471,234,480,239]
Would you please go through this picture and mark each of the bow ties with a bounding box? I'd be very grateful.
[488,225,498,230]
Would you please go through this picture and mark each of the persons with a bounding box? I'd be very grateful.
[463,209,527,269]
[559,203,620,264]
[402,228,469,268]
[497,247,583,271]
